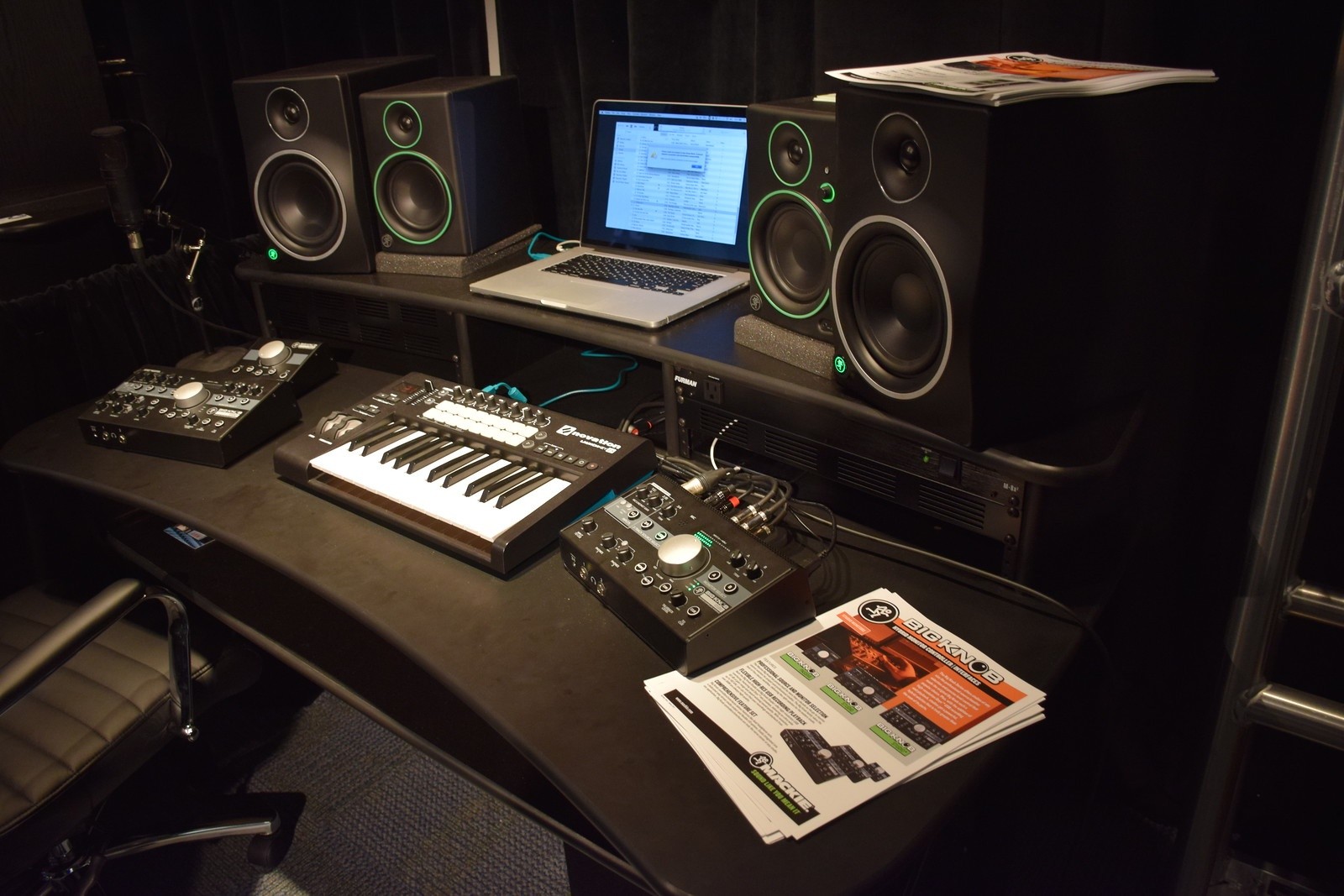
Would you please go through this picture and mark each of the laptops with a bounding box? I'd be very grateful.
[468,98,749,330]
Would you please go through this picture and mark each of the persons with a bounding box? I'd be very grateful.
[883,654,919,688]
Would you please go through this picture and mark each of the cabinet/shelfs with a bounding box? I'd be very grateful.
[0,240,1163,896]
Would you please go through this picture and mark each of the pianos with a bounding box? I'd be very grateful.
[273,368,658,579]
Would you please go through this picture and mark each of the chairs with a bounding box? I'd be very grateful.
[0,578,286,896]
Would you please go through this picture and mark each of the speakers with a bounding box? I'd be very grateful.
[357,74,537,257]
[231,53,426,275]
[833,73,1211,454]
[745,91,836,347]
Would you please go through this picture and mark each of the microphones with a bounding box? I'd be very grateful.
[90,125,149,268]
